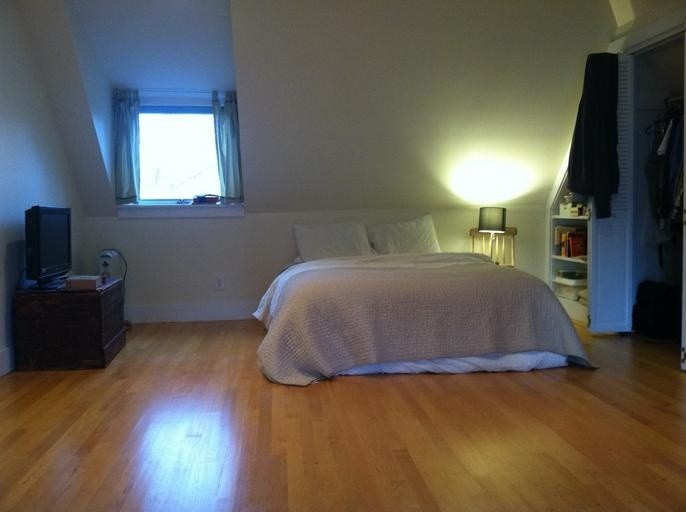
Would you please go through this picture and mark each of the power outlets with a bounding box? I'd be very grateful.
[215,274,225,290]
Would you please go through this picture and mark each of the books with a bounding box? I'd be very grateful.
[553,223,588,262]
[65,275,102,291]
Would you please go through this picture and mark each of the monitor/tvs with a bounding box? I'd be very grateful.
[25,206,71,290]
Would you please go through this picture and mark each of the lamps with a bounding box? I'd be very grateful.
[478,207,506,266]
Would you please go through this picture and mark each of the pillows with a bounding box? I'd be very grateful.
[292,221,373,262]
[368,212,442,256]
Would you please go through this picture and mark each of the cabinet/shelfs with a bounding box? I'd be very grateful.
[11,277,126,370]
[545,142,620,338]
[586,6,686,372]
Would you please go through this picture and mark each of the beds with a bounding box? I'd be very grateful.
[277,251,549,375]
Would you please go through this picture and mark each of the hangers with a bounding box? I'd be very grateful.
[646,97,673,135]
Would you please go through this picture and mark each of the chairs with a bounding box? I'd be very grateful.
[470,228,518,267]
